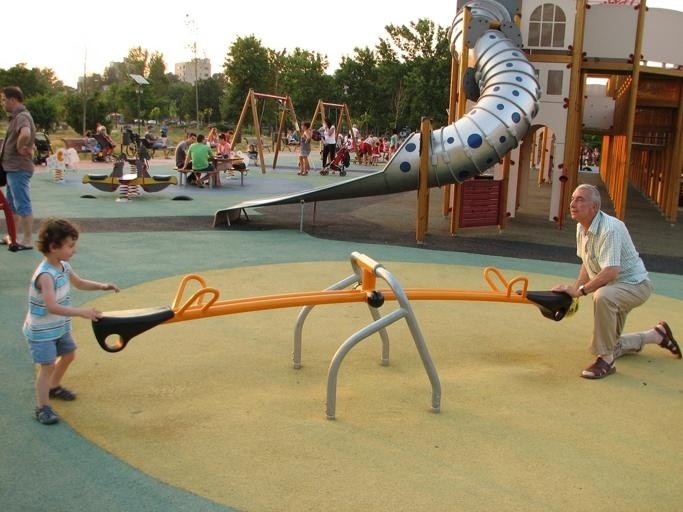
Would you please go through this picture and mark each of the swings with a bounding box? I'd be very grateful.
[248,101,284,155]
[319,107,338,161]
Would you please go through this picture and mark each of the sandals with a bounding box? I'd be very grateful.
[653,320,682,360]
[48,385,76,400]
[33,404,61,426]
[578,355,618,379]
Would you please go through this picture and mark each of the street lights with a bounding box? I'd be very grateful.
[129,71,151,139]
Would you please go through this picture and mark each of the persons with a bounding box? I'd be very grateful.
[22,217,120,425]
[592,148,599,168]
[0,87,37,250]
[551,184,682,379]
[583,148,590,166]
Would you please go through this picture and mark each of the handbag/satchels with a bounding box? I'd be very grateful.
[0,158,8,188]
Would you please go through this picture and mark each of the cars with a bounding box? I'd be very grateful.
[135,118,198,128]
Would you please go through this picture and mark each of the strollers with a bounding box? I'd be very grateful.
[31,131,54,167]
[92,133,114,163]
[319,144,350,176]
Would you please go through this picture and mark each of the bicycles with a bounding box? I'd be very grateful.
[127,130,156,160]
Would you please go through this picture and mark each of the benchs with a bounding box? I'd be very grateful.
[173,167,219,189]
[59,137,93,155]
[281,137,297,153]
[138,136,176,160]
[225,168,250,186]
[242,135,271,154]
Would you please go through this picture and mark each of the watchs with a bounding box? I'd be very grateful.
[578,285,588,296]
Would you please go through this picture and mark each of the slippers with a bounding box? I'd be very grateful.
[8,244,34,251]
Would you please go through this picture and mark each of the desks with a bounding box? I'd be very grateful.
[206,157,244,186]
